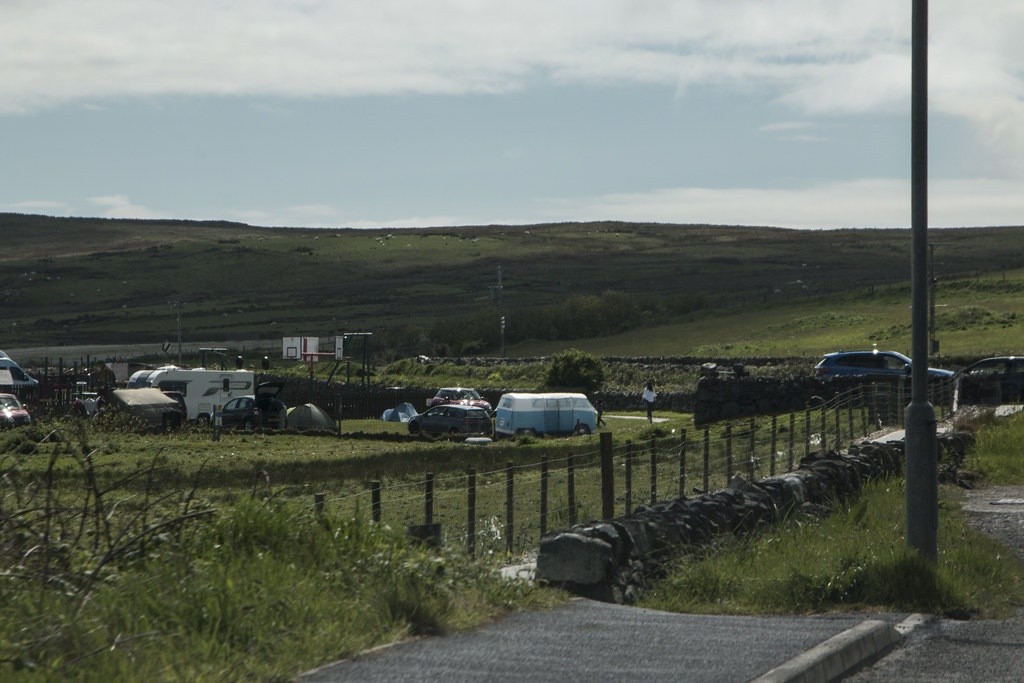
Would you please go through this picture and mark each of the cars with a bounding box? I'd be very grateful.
[432,386,494,417]
[940,355,1024,404]
[0,393,31,429]
[210,394,289,432]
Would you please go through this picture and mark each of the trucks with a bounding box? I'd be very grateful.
[125,365,257,429]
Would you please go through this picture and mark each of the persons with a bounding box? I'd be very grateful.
[642,383,657,417]
[597,399,606,425]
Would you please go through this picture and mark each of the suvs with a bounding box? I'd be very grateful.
[407,404,493,444]
[813,350,956,386]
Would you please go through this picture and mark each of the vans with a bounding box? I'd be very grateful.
[495,392,597,439]
